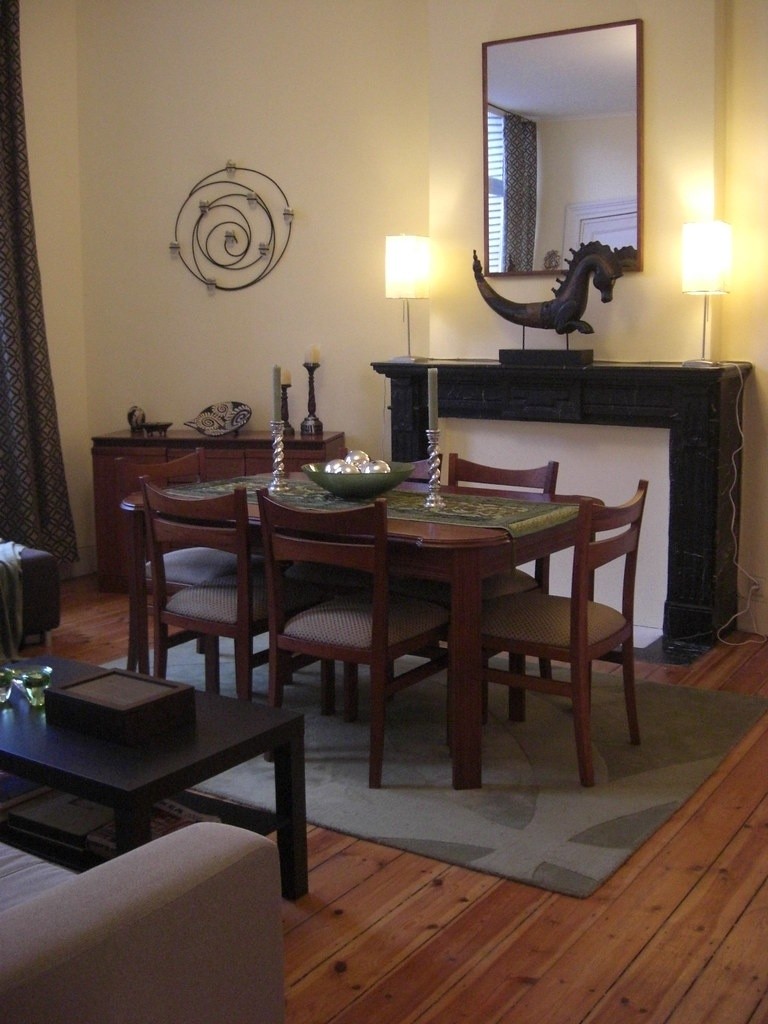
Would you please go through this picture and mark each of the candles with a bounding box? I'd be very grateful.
[303,339,320,363]
[271,364,283,420]
[281,367,292,384]
[426,365,438,428]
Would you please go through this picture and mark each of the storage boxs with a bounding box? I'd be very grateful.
[44,668,195,740]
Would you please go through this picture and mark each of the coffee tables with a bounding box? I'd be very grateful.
[1,655,312,901]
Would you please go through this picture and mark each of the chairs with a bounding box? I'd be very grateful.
[111,447,263,659]
[285,443,441,590]
[439,479,649,783]
[254,490,453,790]
[136,476,336,714]
[446,453,559,725]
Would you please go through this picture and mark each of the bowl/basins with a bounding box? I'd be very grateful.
[300,461,417,499]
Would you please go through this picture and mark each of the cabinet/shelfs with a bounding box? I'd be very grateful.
[89,428,345,588]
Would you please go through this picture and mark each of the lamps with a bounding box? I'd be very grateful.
[680,220,730,367]
[385,234,435,361]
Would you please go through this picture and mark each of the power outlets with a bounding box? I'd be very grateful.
[744,576,766,604]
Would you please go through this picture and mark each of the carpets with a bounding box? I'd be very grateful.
[102,631,767,899]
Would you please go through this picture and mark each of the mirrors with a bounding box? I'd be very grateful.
[480,19,644,278]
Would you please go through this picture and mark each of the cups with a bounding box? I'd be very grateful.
[1,667,17,703]
[21,666,51,706]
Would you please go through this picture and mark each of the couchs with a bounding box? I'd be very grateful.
[0,537,60,647]
[1,816,286,1024]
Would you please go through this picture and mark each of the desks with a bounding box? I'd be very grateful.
[166,472,605,791]
